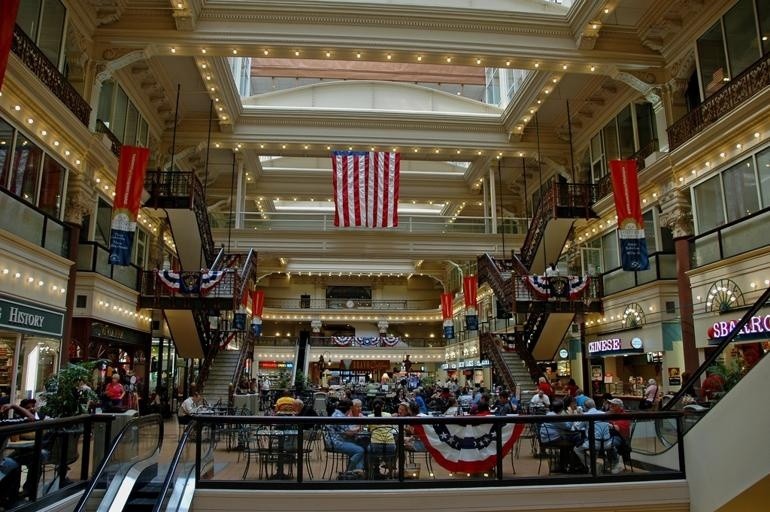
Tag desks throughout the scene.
[6,441,42,505]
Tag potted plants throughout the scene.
[39,354,111,461]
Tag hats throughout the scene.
[607,397,624,407]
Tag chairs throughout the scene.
[175,373,678,481]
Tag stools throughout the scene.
[19,460,56,499]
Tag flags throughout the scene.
[332,150,401,228]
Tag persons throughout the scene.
[643,378,658,403]
[105,373,126,406]
[292,398,322,440]
[681,372,697,397]
[542,261,560,278]
[228,374,271,410]
[177,391,202,424]
[274,388,297,414]
[9,398,47,502]
[0,397,36,509]
[698,367,727,403]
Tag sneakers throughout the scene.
[612,464,624,474]
